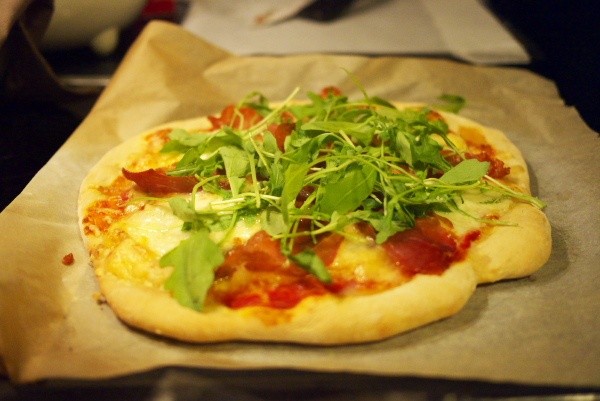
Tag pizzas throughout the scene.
[77,99,552,346]
